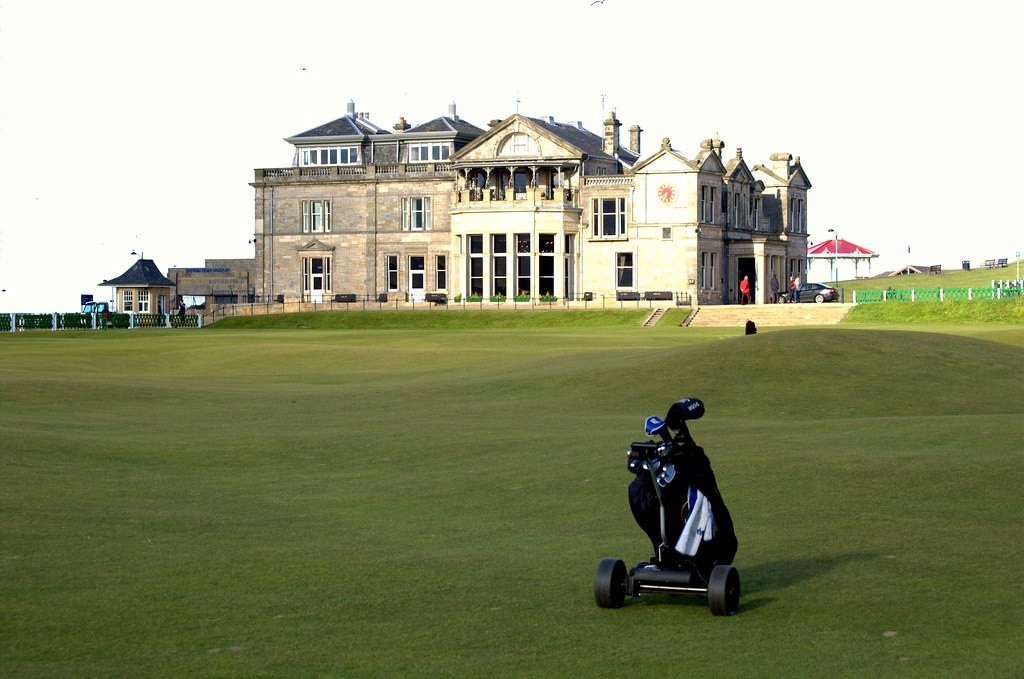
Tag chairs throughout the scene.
[273,295,284,303]
[375,294,387,302]
[581,292,592,301]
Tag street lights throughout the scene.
[828,229,839,302]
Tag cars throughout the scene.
[770,282,840,303]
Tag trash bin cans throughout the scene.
[962,261,970,271]
[277,295,284,304]
[249,293,255,303]
[379,293,387,302]
[584,292,592,301]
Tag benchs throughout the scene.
[616,291,640,300]
[332,294,356,302]
[424,293,447,304]
[645,292,672,300]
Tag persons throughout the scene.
[158,301,162,325]
[770,274,780,304]
[740,276,751,304]
[177,299,187,329]
[102,305,109,330]
[789,275,803,303]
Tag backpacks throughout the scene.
[745,321,757,335]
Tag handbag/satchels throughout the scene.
[629,443,738,589]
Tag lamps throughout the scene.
[249,239,256,243]
